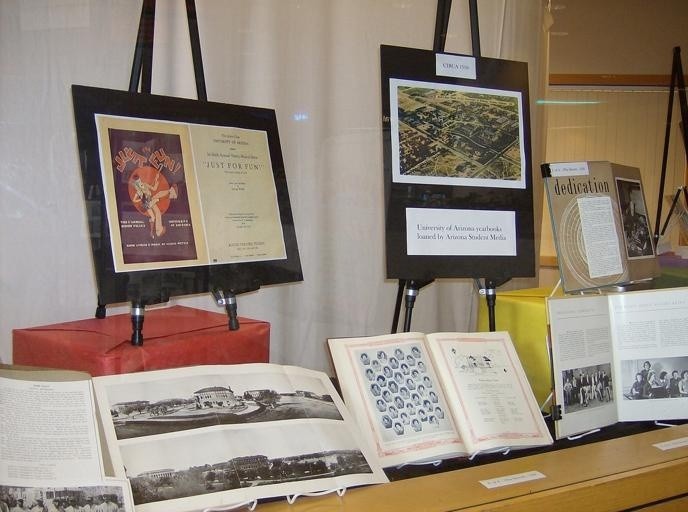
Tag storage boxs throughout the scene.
[12,305,271,378]
[476,285,615,415]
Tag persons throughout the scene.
[130,173,179,238]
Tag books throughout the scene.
[540,160,663,295]
[545,287,688,442]
[326,329,554,470]
[0,361,389,511]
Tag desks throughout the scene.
[229,424,688,512]
